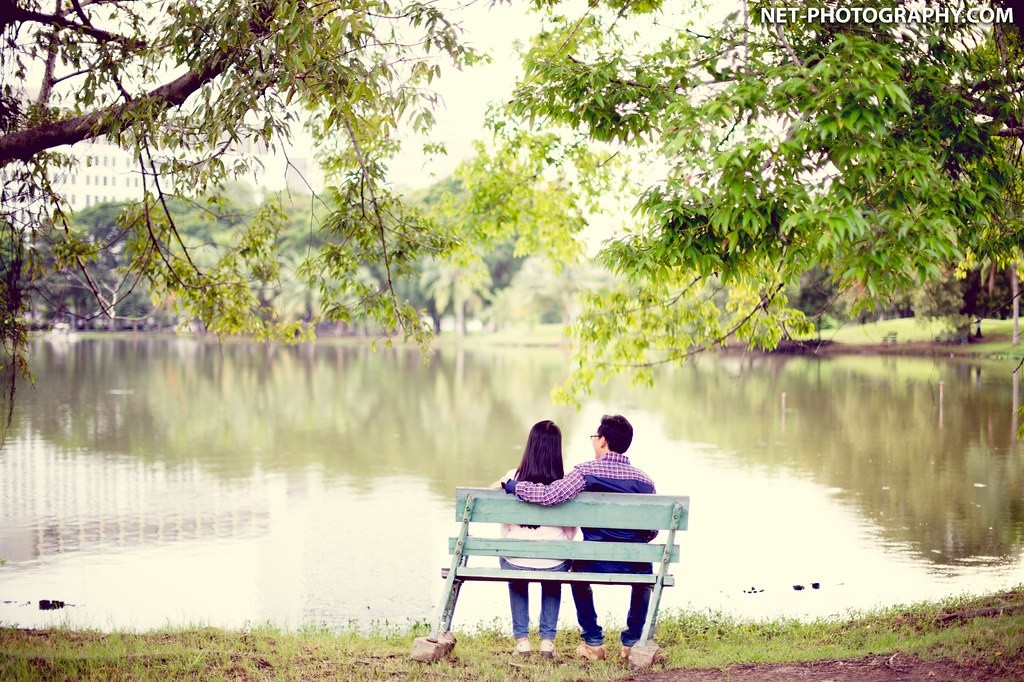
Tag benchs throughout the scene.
[424,487,689,645]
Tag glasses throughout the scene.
[590,435,601,441]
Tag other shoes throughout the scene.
[621,646,631,659]
[577,642,606,662]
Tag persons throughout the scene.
[489,420,584,658]
[493,414,660,660]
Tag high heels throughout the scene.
[540,640,557,660]
[511,641,530,662]
